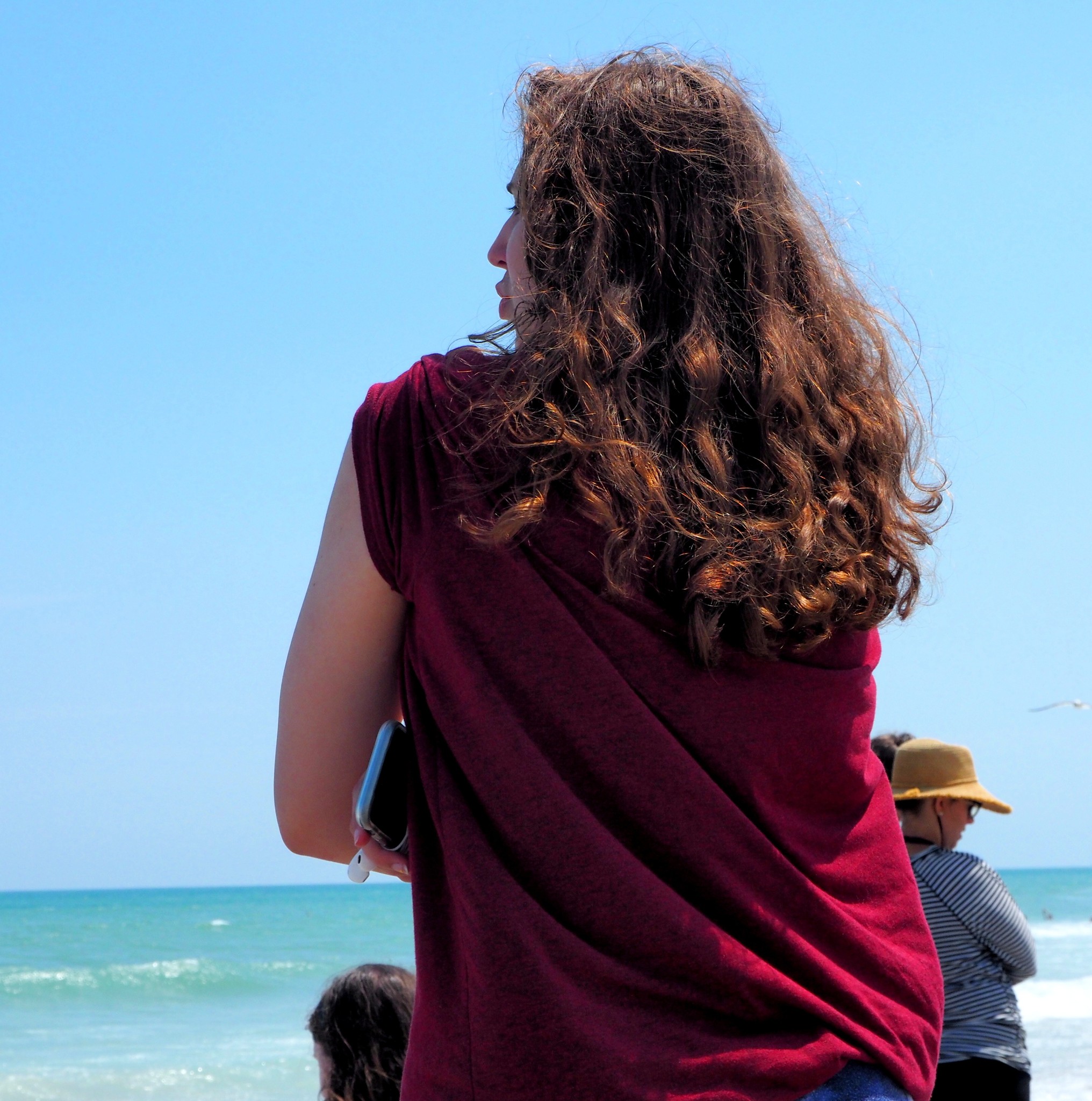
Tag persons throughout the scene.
[870,733,915,784]
[886,737,1036,1101]
[272,51,943,1100]
[305,964,418,1101]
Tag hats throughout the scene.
[889,738,1013,815]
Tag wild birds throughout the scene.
[1024,698,1092,714]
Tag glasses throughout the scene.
[966,800,983,817]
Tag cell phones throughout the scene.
[356,720,411,856]
